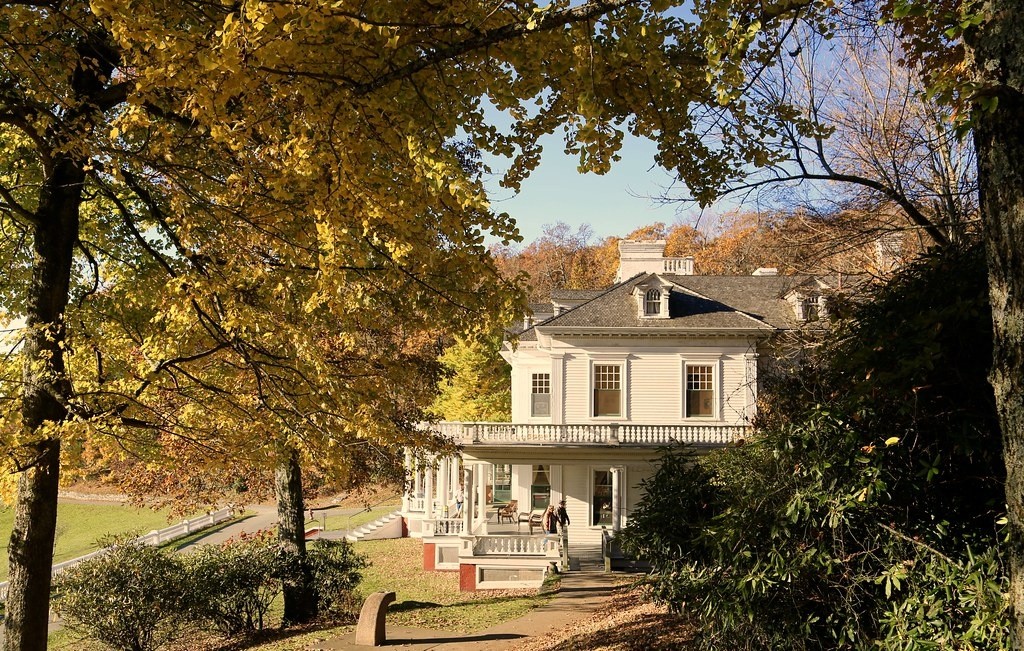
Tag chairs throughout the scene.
[498,499,518,524]
[517,508,547,534]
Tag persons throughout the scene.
[455,484,463,512]
[555,500,570,527]
[542,505,561,548]
